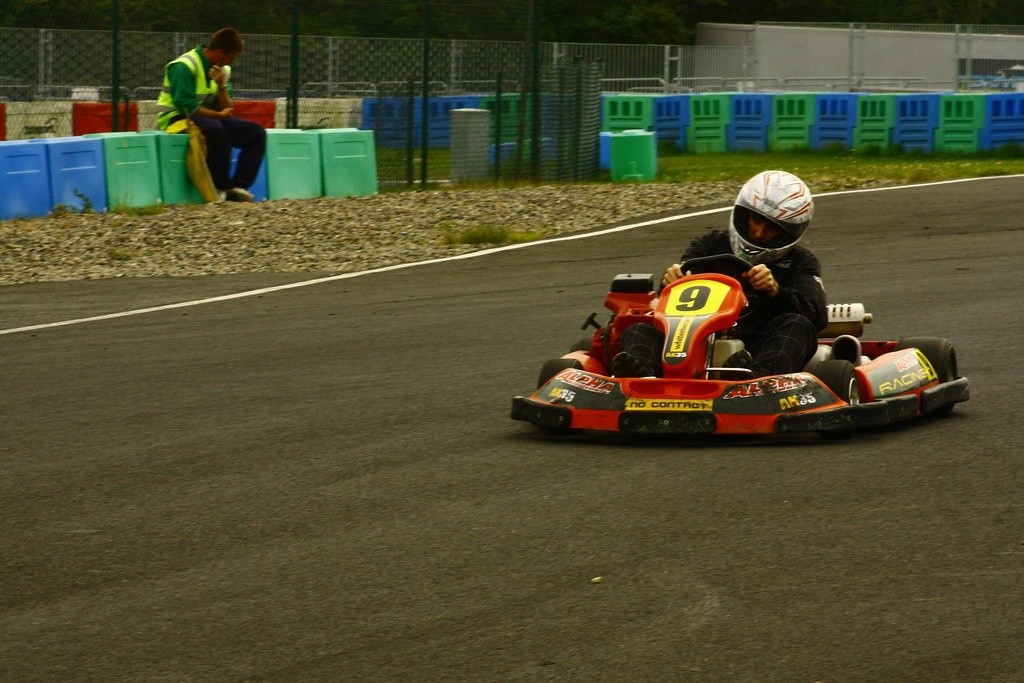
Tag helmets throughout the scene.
[729,170,814,265]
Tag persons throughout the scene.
[610,170,828,379]
[156,27,267,204]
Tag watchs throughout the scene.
[219,84,228,92]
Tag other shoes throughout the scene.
[611,352,644,378]
[720,349,752,380]
[214,192,226,203]
[228,187,253,202]
